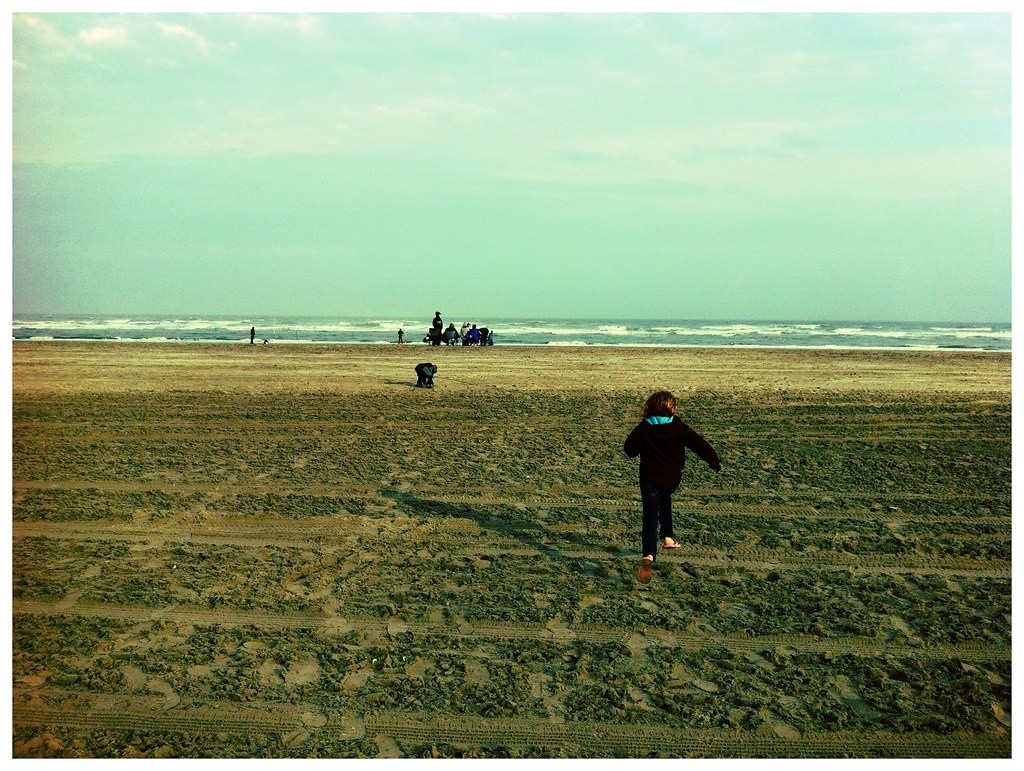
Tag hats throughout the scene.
[467,323,470,325]
[436,312,441,314]
[463,322,467,327]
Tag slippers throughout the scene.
[661,540,681,549]
[636,557,653,584]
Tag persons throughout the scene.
[264,340,268,344]
[623,391,721,584]
[424,312,493,346]
[398,329,404,344]
[415,363,437,388]
[250,327,255,344]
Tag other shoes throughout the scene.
[426,383,433,387]
[416,381,423,386]
